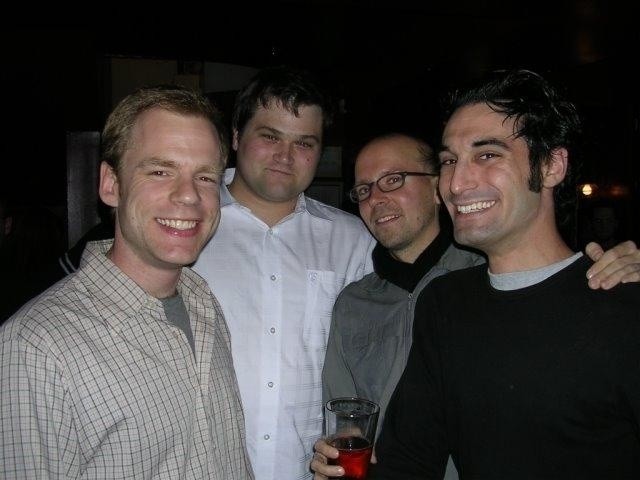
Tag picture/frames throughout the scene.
[304,180,348,213]
[315,141,344,179]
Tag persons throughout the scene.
[320,125,639,480]
[0,86,254,480]
[191,71,377,480]
[310,67,639,480]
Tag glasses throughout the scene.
[347,171,432,203]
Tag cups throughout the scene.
[323,396,380,480]
